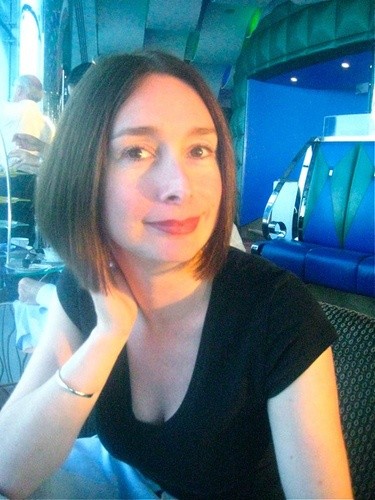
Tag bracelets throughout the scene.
[38,157,44,167]
[54,369,95,398]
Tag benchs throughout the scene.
[250,134,375,300]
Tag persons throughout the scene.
[0,50,353,500]
[3,74,56,223]
[7,62,95,169]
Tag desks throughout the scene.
[4,253,71,305]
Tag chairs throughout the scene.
[295,298,375,499]
